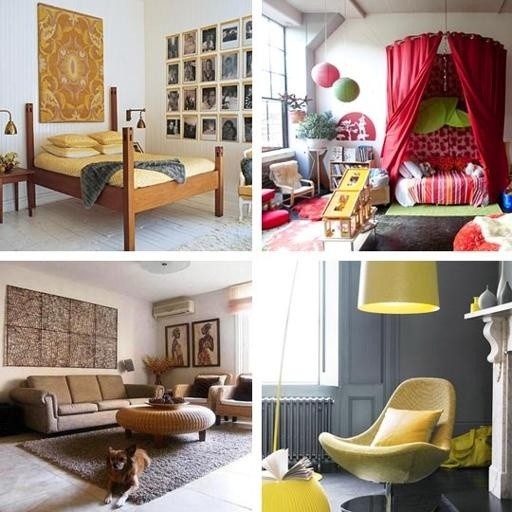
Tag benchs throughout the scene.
[267,159,312,210]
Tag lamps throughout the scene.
[0,109,17,136]
[310,0,340,89]
[356,261,440,316]
[332,0,360,103]
[126,107,146,128]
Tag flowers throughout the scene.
[141,353,173,374]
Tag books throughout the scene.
[262,446,313,483]
[328,144,370,189]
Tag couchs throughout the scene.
[215,373,252,424]
[174,372,232,421]
[11,374,165,435]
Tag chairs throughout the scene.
[239,148,252,222]
[318,377,456,512]
[366,170,391,207]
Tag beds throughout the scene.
[24,88,224,251]
[380,32,508,206]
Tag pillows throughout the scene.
[90,131,122,143]
[41,144,101,159]
[193,377,220,398]
[94,145,127,154]
[234,377,252,401]
[397,165,411,179]
[47,134,99,148]
[371,408,442,449]
[404,160,422,178]
[240,157,251,186]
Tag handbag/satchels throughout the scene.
[440,426,492,468]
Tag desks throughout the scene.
[262,466,330,512]
[0,167,36,224]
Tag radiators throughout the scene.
[263,396,335,471]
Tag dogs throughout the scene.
[103,443,151,507]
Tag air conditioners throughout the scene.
[153,300,195,318]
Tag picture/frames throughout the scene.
[133,142,142,153]
[192,318,221,367]
[165,323,190,369]
[165,15,253,145]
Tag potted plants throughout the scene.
[279,93,312,122]
[294,111,338,149]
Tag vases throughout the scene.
[155,375,162,385]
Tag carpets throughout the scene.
[290,196,329,222]
[358,213,485,251]
[20,422,253,505]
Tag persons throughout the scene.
[197,323,215,364]
[169,326,185,365]
[167,19,251,143]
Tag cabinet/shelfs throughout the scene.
[330,159,374,192]
[318,168,379,250]
